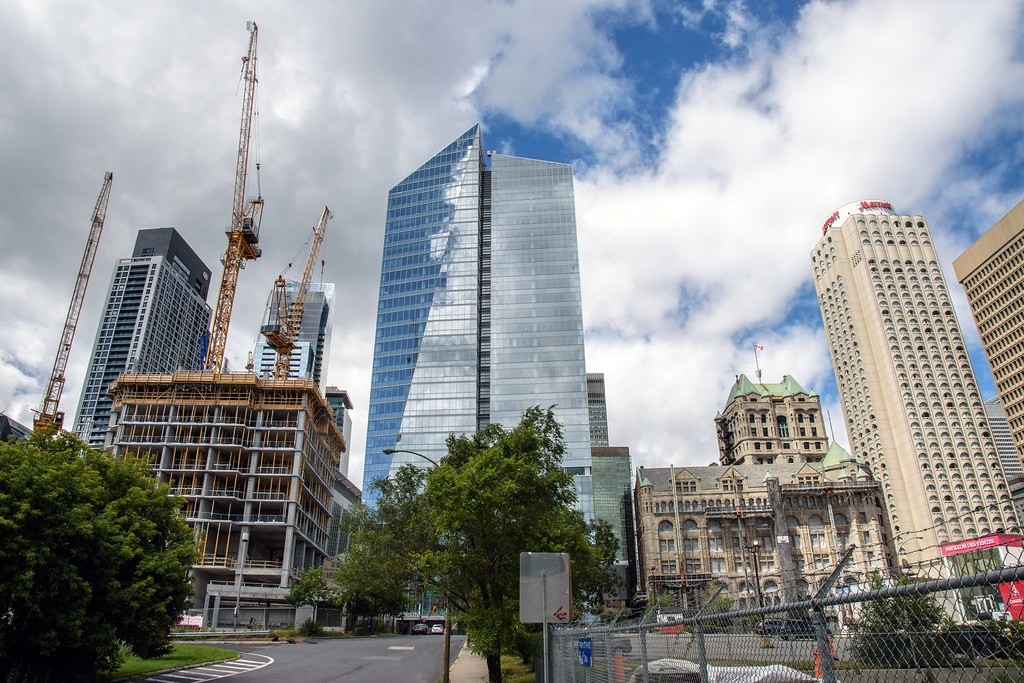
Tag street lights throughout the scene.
[741,531,775,648]
[382,447,452,683]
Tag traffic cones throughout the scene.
[828,636,839,660]
[815,652,825,683]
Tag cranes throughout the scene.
[260,205,334,380]
[198,18,266,374]
[27,171,117,436]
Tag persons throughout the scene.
[810,629,836,683]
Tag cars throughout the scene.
[412,623,429,634]
[431,624,445,635]
[566,636,632,657]
[783,619,834,640]
[755,619,785,635]
